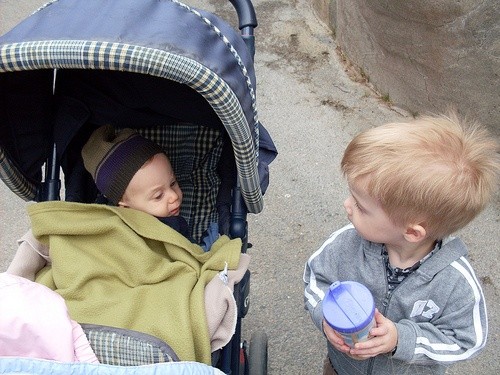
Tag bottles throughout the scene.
[323,281,377,360]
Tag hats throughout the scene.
[81,123,164,205]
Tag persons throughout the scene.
[300,103,500,375]
[53,121,199,366]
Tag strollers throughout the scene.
[0,0,279,375]
